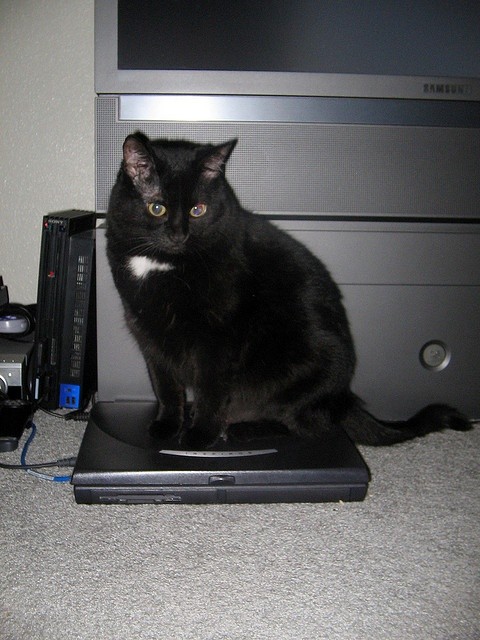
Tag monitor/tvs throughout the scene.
[93,1,480,407]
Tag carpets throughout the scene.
[0,400,480,640]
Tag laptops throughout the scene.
[65,403,373,506]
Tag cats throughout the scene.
[103,128,475,453]
